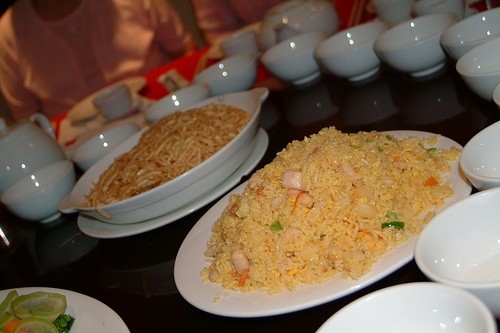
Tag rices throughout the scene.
[199,126,461,302]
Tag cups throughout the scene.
[221,31,260,60]
[373,0,465,23]
[92,84,132,118]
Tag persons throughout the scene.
[0,0,194,129]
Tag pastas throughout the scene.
[83,102,250,219]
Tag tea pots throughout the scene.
[1,113,63,191]
[257,0,339,51]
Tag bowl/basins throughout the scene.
[440,7,499,60]
[260,32,325,85]
[374,14,456,78]
[58,88,270,223]
[193,54,258,92]
[1,161,74,224]
[72,122,138,172]
[144,82,211,121]
[459,120,500,192]
[315,282,498,333]
[312,18,387,81]
[456,35,499,106]
[415,186,500,316]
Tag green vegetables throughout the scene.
[53,314,75,333]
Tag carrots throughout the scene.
[5,320,28,333]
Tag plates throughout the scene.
[77,127,269,239]
[0,288,129,333]
[67,76,147,117]
[173,130,472,317]
